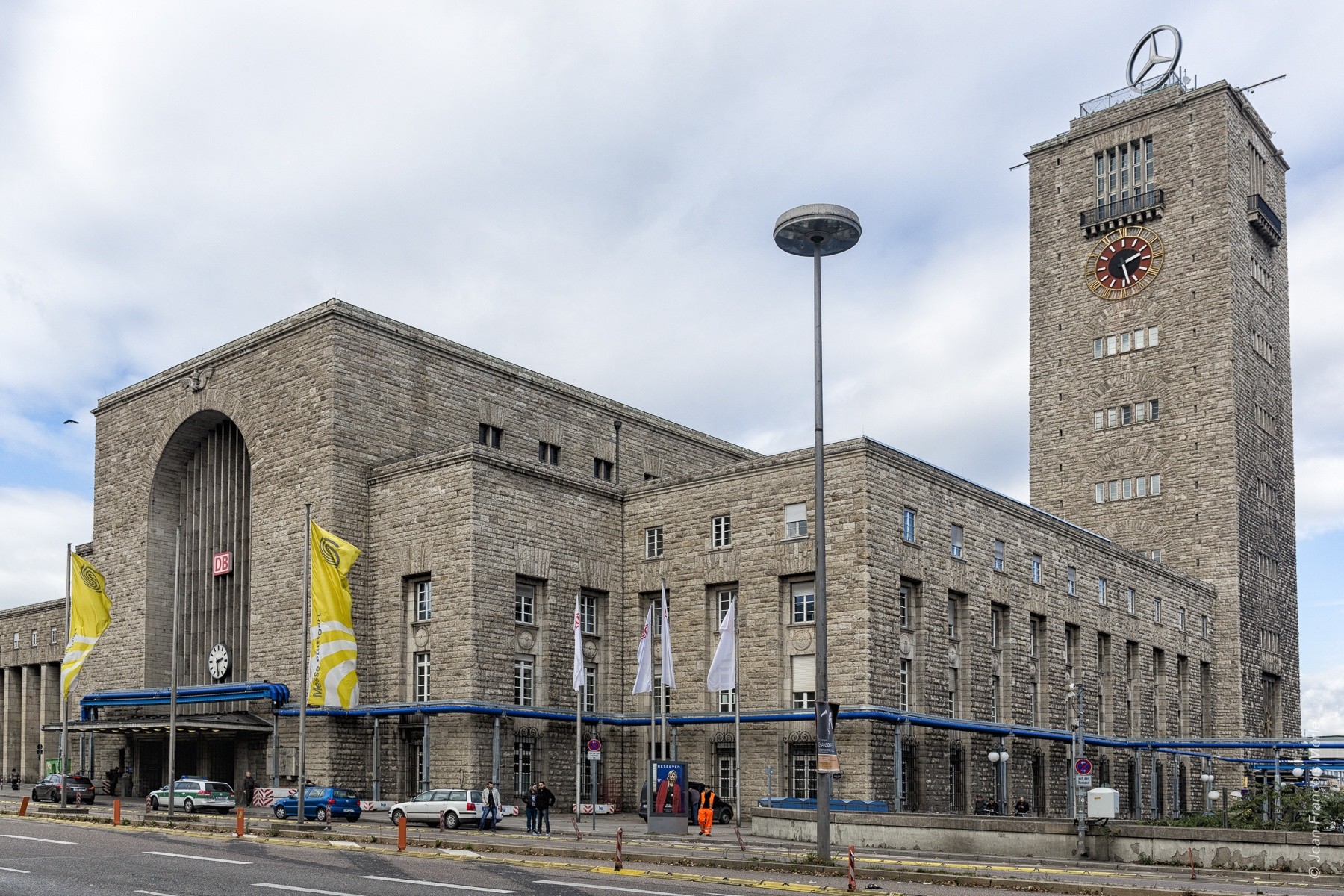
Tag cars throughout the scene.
[273,786,361,822]
[148,774,235,813]
[387,789,504,830]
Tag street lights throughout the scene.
[1199,760,1220,816]
[774,203,864,861]
[1293,767,1324,791]
[1207,787,1242,828]
[985,741,1010,815]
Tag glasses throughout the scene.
[533,786,537,789]
[539,784,544,786]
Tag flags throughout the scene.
[305,518,361,710]
[572,593,585,693]
[60,551,113,702]
[706,596,736,691]
[631,604,653,695]
[661,582,677,689]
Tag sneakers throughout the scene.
[532,830,537,836]
[491,829,496,833]
[536,831,542,835]
[707,834,712,837]
[478,830,484,834]
[699,831,704,835]
[546,832,550,836]
[525,829,531,834]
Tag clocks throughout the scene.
[1082,225,1164,300]
[207,644,232,680]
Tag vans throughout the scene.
[638,780,733,825]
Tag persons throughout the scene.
[108,767,121,797]
[477,781,503,834]
[973,793,1029,817]
[533,781,555,836]
[305,779,315,787]
[689,788,701,825]
[697,785,717,837]
[242,771,260,809]
[522,783,538,834]
[656,770,682,813]
[10,769,21,790]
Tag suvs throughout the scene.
[31,772,95,804]
